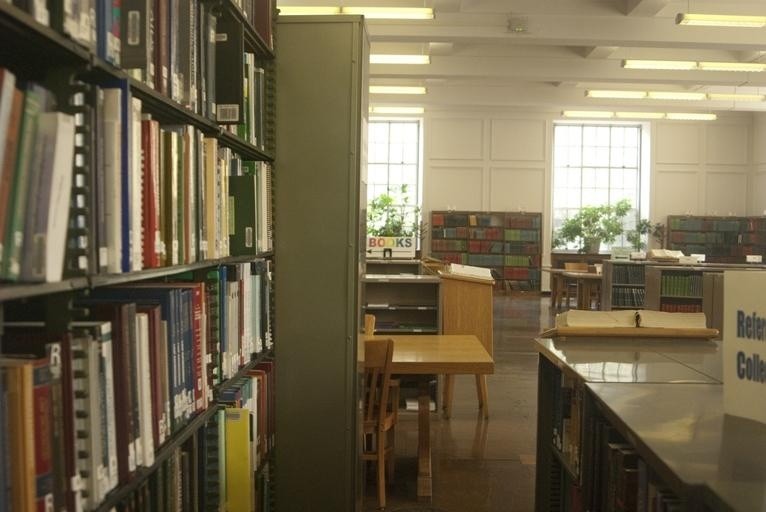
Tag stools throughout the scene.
[443,374,488,419]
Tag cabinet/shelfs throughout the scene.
[601,261,766,311]
[432,211,542,290]
[667,215,766,264]
[534,339,766,512]
[362,274,442,416]
[646,266,766,313]
[0,0,275,512]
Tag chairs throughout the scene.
[365,314,399,425]
[565,262,587,307]
[364,340,395,509]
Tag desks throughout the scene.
[562,272,602,310]
[542,268,588,308]
[422,260,496,362]
[359,334,493,505]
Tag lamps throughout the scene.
[561,0,766,120]
[276,0,435,115]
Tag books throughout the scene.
[432,214,540,291]
[367,300,436,411]
[612,216,766,312]
[1,0,275,150]
[1,71,274,283]
[547,366,688,512]
[0,257,275,511]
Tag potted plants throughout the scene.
[552,199,632,254]
